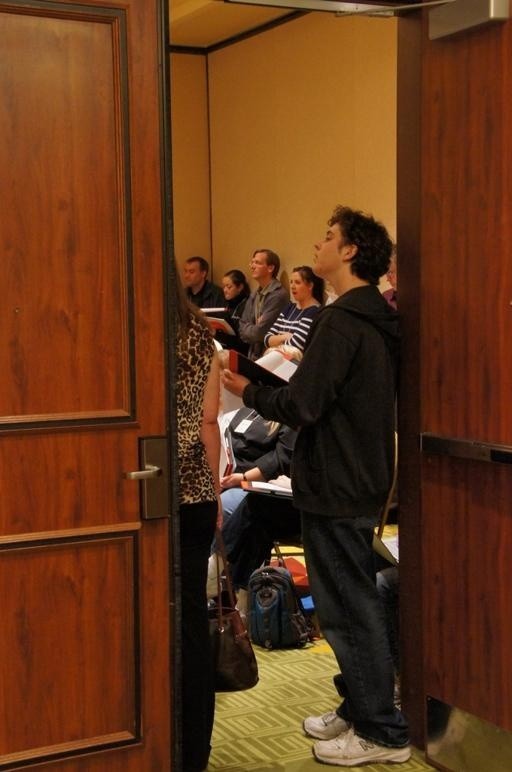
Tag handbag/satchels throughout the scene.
[207,608,259,693]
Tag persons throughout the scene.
[211,203,417,768]
[169,258,224,770]
[384,250,398,308]
[185,250,325,596]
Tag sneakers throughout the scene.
[206,571,224,599]
[311,728,412,767]
[302,710,354,740]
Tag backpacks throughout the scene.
[247,565,311,650]
